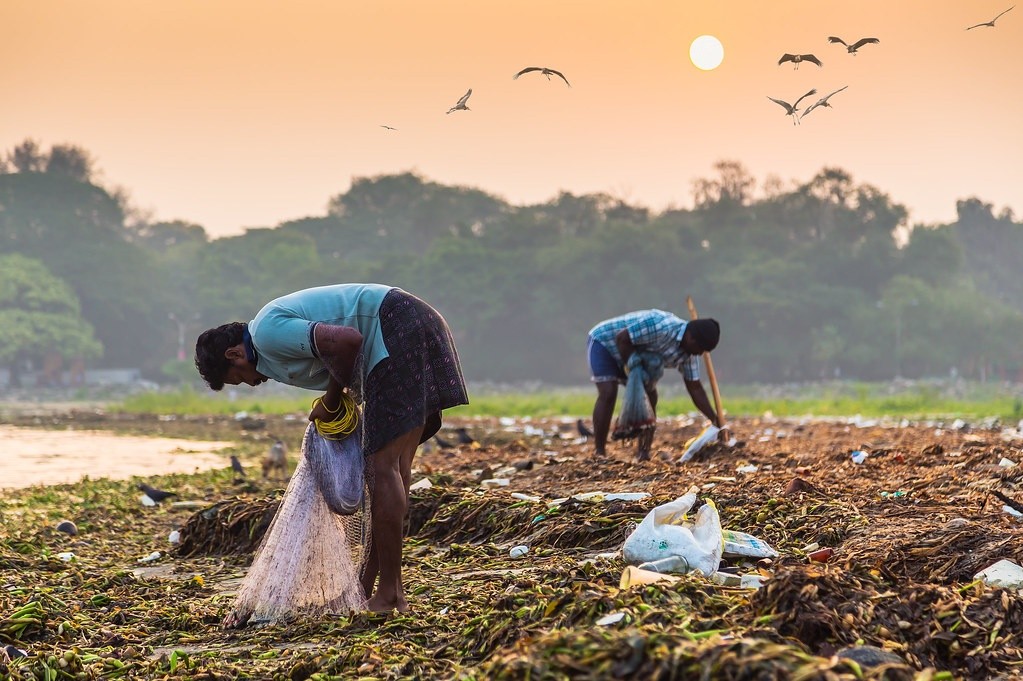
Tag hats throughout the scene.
[688,318,721,352]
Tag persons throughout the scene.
[194,282,470,619]
[586,307,730,462]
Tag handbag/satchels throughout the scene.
[623,489,725,579]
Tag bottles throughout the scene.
[639,555,690,576]
[800,548,834,565]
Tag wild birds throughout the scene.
[767,86,849,125]
[230,455,247,481]
[828,35,880,56]
[139,482,178,506]
[381,124,397,132]
[446,89,473,114]
[578,419,594,443]
[434,427,473,447]
[514,67,572,88]
[963,5,1015,30]
[778,53,824,71]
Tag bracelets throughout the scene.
[319,393,342,414]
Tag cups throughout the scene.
[618,565,684,593]
